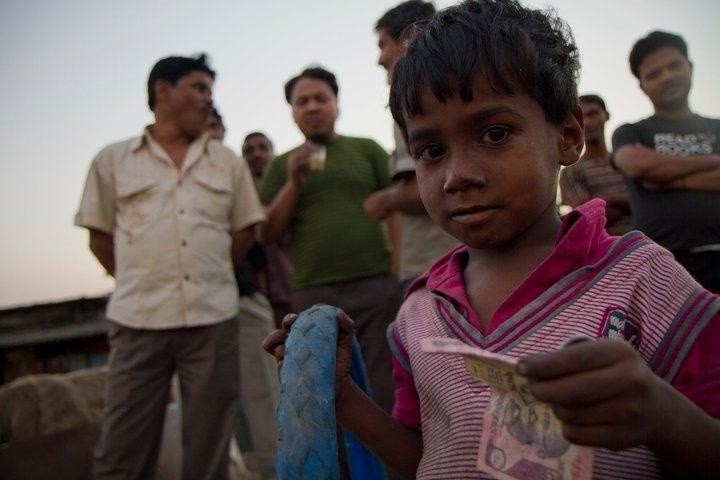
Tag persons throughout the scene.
[262,0,720,480]
[259,64,403,418]
[363,1,462,302]
[170,109,256,480]
[242,132,275,185]
[611,30,720,293]
[560,94,632,229]
[74,55,268,480]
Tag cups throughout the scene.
[306,146,325,171]
